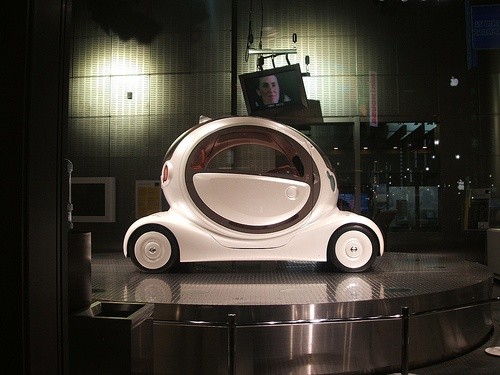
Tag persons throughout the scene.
[254,74,291,107]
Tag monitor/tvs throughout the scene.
[238,63,308,118]
[71,176,116,222]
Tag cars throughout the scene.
[124,115,385,275]
